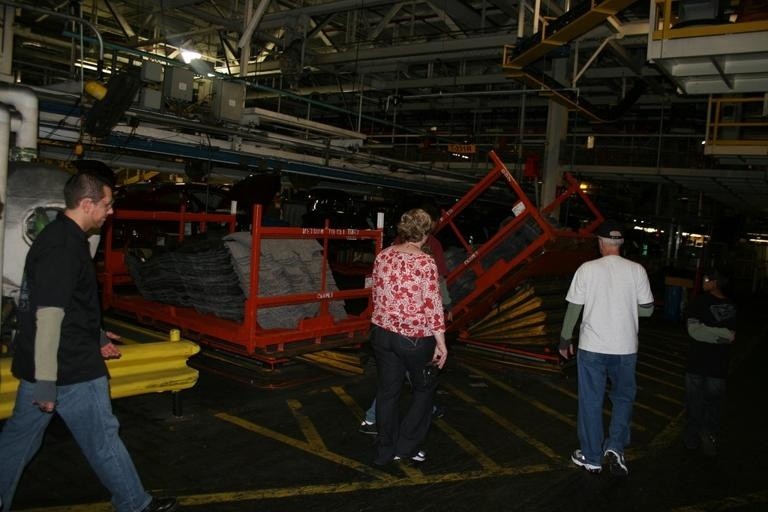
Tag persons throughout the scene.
[1,166,180,512]
[682,264,740,459]
[370,207,449,465]
[557,218,657,476]
[360,206,448,436]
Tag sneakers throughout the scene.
[358,420,378,435]
[686,431,722,451]
[412,450,427,465]
[142,496,177,512]
[571,449,603,473]
[604,449,628,478]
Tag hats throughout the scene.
[590,221,625,240]
